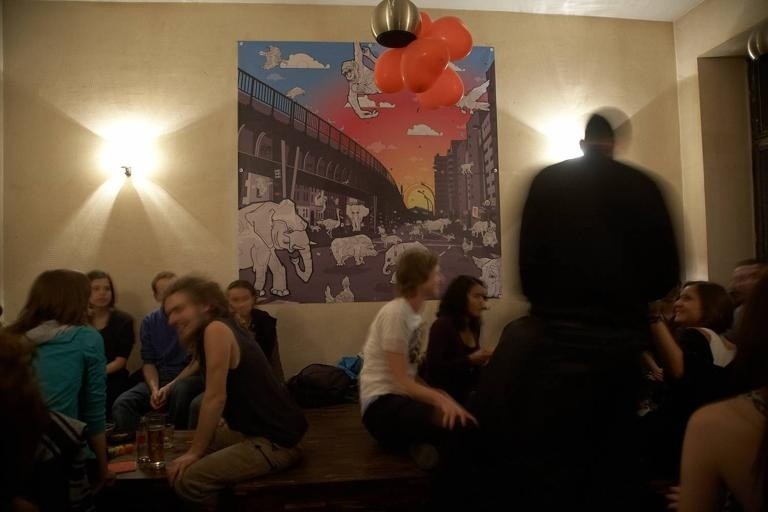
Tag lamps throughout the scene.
[371,0,423,50]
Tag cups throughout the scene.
[135,413,175,471]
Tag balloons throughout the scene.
[374,10,473,110]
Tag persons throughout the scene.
[634,256,768,512]
[362,251,530,512]
[0,268,308,512]
[517,109,682,512]
